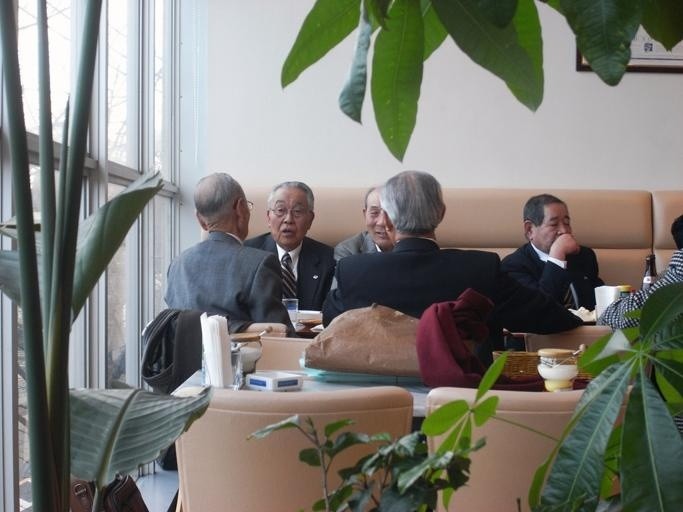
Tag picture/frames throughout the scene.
[573,22,682,71]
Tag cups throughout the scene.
[199,352,241,390]
[227,332,263,377]
[594,304,608,320]
[536,348,579,392]
[280,299,298,320]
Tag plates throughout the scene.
[297,319,321,327]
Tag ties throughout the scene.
[281,254,296,298]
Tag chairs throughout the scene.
[159,382,415,512]
[423,384,626,512]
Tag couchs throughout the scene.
[648,190,682,275]
[196,181,653,295]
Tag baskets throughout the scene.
[491,351,593,382]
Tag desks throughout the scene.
[164,320,629,418]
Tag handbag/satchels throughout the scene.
[305,305,419,375]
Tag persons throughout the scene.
[595,210,682,439]
[163,173,305,338]
[331,185,396,290]
[241,179,335,312]
[498,193,605,324]
[319,169,585,371]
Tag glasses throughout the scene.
[272,206,305,216]
[243,200,252,210]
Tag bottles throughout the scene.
[640,254,658,291]
[501,331,522,352]
[617,285,632,299]
